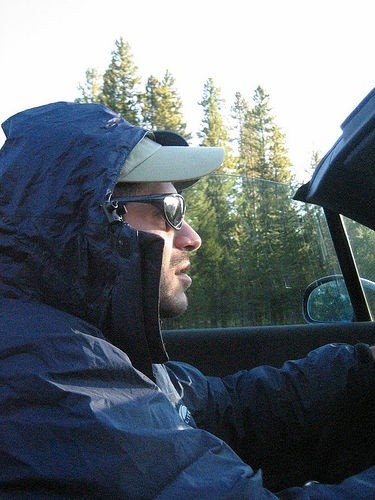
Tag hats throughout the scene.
[118,138,225,190]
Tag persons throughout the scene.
[0,100,375,500]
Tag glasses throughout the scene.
[119,192,185,230]
